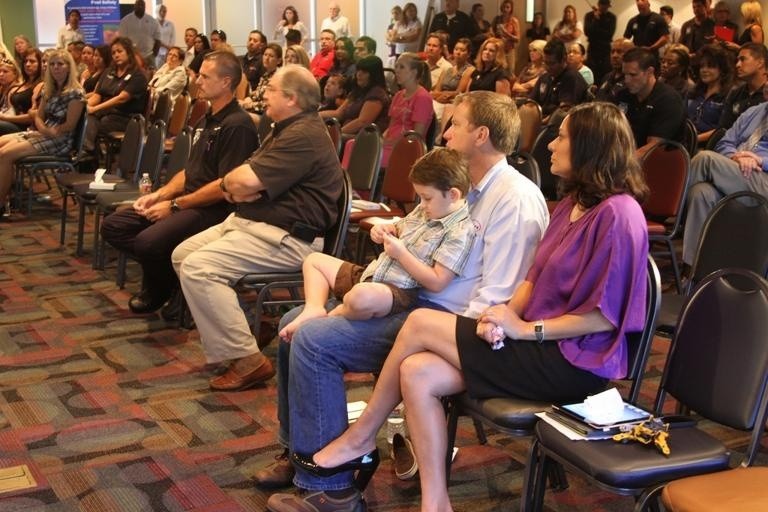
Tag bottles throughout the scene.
[386,402,405,445]
[138,173,152,199]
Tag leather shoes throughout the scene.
[161,288,182,318]
[208,356,276,392]
[250,321,276,351]
[128,288,171,313]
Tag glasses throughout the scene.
[262,85,285,93]
[543,60,558,66]
[217,28,221,35]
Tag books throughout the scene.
[546,409,621,437]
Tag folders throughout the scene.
[542,405,667,437]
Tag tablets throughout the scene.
[552,397,655,429]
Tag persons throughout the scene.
[705,2,739,46]
[291,101,647,512]
[527,38,595,102]
[680,0,714,49]
[120,1,159,56]
[254,91,550,511]
[619,46,688,139]
[330,35,355,71]
[286,45,310,66]
[251,43,283,115]
[555,4,584,49]
[375,51,433,167]
[172,63,345,391]
[209,29,232,51]
[317,71,351,111]
[725,1,763,52]
[385,6,403,60]
[309,29,337,79]
[619,42,619,45]
[427,0,472,48]
[685,46,732,150]
[345,33,377,75]
[284,27,303,45]
[241,29,268,93]
[720,42,768,139]
[589,38,637,104]
[466,3,493,37]
[466,37,511,93]
[320,56,390,134]
[67,39,85,67]
[273,5,308,46]
[512,42,549,101]
[416,32,452,87]
[0,50,86,210]
[82,45,113,99]
[623,0,666,53]
[187,33,207,68]
[660,44,695,102]
[78,36,149,168]
[213,43,235,55]
[277,146,476,344]
[39,48,55,74]
[11,33,34,55]
[153,4,176,50]
[320,1,352,37]
[390,3,422,62]
[79,44,96,87]
[101,50,256,313]
[182,29,200,48]
[492,0,520,80]
[57,9,86,57]
[523,12,550,40]
[430,38,474,99]
[662,72,767,296]
[146,46,188,113]
[567,42,594,88]
[659,5,680,54]
[583,0,618,69]
[0,53,23,112]
[0,47,43,133]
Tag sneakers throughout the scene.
[393,433,418,480]
[266,488,368,512]
[254,453,293,486]
[72,148,96,161]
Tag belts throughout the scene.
[316,230,326,237]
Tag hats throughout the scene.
[598,0,611,7]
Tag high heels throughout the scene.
[292,447,381,492]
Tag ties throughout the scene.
[736,115,768,152]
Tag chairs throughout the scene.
[633,141,690,296]
[344,131,428,265]
[325,117,342,158]
[532,267,768,512]
[11,98,87,216]
[662,384,768,512]
[56,114,132,255]
[78,113,145,266]
[120,125,194,289]
[446,255,660,512]
[384,67,399,96]
[508,152,540,193]
[347,124,382,261]
[237,170,352,347]
[516,97,542,156]
[653,191,768,337]
[99,119,166,269]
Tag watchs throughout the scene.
[533,318,545,345]
[169,198,178,214]
